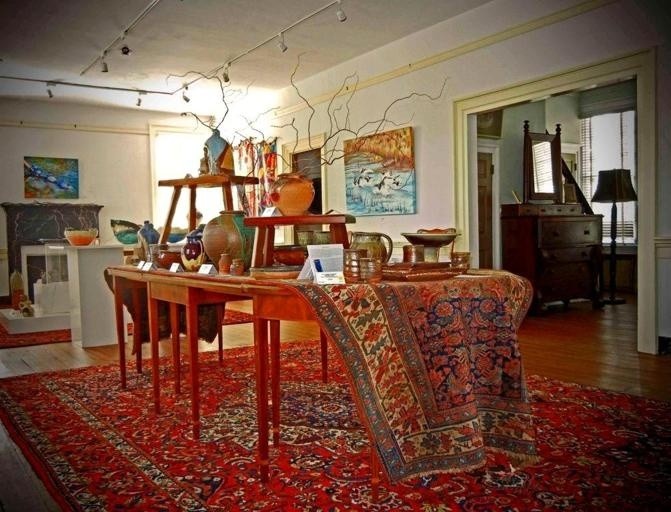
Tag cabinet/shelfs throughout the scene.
[502,203,602,317]
[67,246,128,349]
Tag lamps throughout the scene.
[46,83,145,106]
[591,169,637,304]
[100,33,130,72]
[183,3,347,102]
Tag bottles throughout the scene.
[229,258,244,274]
[217,253,231,275]
[124,251,134,265]
[9,268,23,310]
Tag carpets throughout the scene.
[0,308,254,348]
[0,339,671,512]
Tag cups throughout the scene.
[453,252,470,273]
[360,258,381,283]
[342,247,367,276]
[401,245,425,263]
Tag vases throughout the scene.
[138,130,315,272]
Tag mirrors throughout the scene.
[523,120,562,205]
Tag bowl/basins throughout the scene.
[273,244,305,264]
[160,250,180,269]
[65,230,96,246]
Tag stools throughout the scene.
[245,215,356,267]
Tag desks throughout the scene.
[103,266,540,502]
